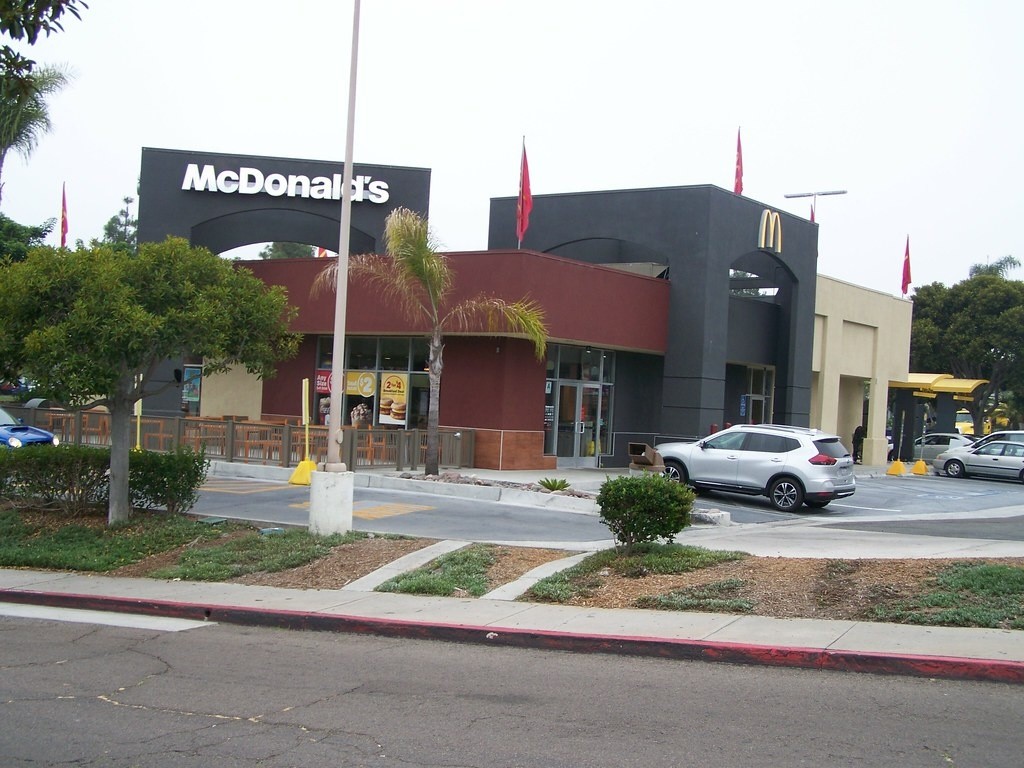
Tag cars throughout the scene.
[0,407,61,453]
[933,440,1024,484]
[885,403,1024,463]
[0,374,42,396]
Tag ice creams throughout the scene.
[351,404,372,429]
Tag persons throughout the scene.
[852,424,867,465]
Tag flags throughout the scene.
[60,181,68,248]
[811,207,814,222]
[901,233,912,294]
[515,145,533,241]
[735,124,743,195]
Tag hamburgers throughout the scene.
[379,397,393,415]
[390,402,406,419]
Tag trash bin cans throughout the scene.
[24,398,50,431]
[628,443,665,480]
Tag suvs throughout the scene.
[652,423,856,513]
